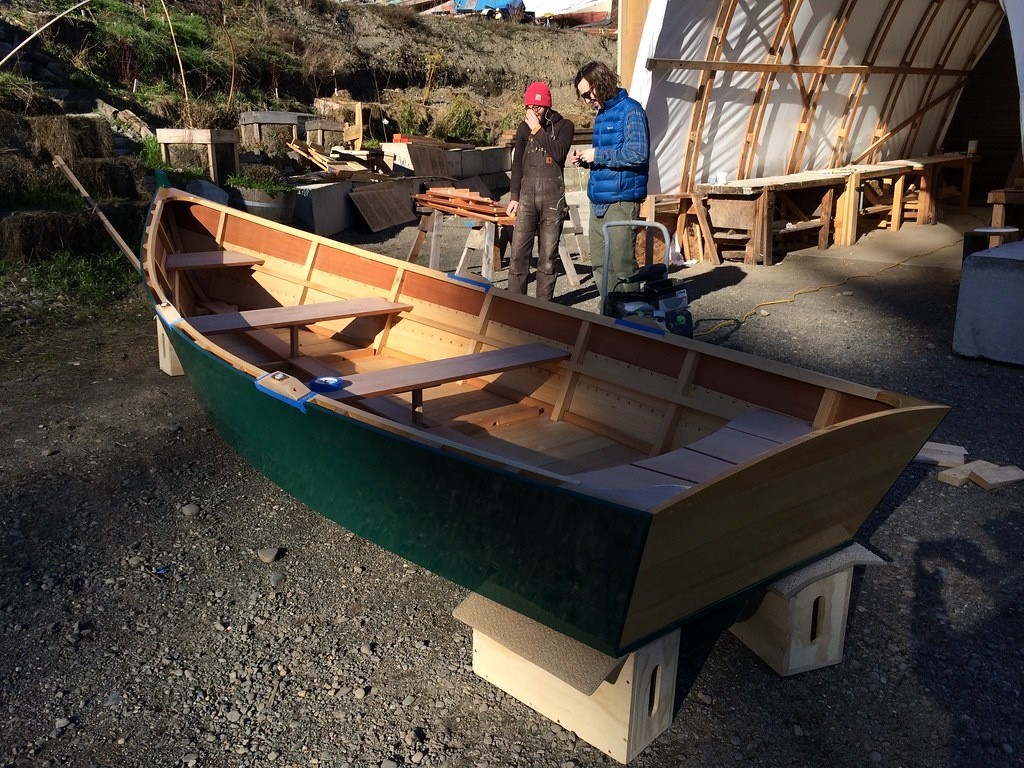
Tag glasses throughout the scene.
[581,85,596,99]
[525,106,540,112]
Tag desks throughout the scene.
[406,193,588,286]
[986,187,1024,248]
[696,151,978,266]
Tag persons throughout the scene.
[570,62,651,298]
[505,82,575,302]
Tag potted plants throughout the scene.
[361,139,384,173]
[223,164,298,224]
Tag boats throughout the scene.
[137,166,953,659]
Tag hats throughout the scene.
[524,82,552,107]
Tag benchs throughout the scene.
[183,296,413,358]
[302,343,572,425]
[165,249,265,270]
[567,410,820,509]
